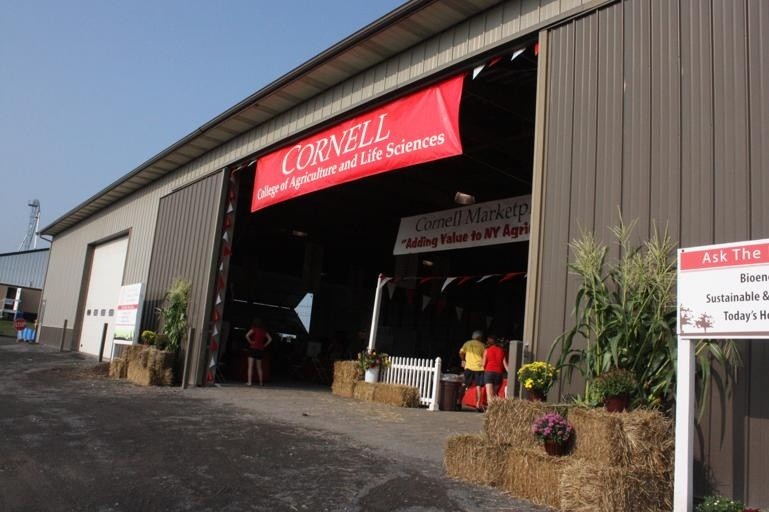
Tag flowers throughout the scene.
[532,412,571,443]
[359,349,388,369]
[517,362,559,392]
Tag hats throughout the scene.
[471,330,483,339]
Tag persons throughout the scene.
[455,329,486,412]
[244,317,273,387]
[481,337,508,408]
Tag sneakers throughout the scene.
[476,404,484,412]
[456,403,461,410]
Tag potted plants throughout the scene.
[592,369,640,412]
[140,330,155,346]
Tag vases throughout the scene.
[545,439,570,454]
[527,391,547,401]
[364,366,380,383]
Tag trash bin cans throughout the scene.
[440,373,465,411]
